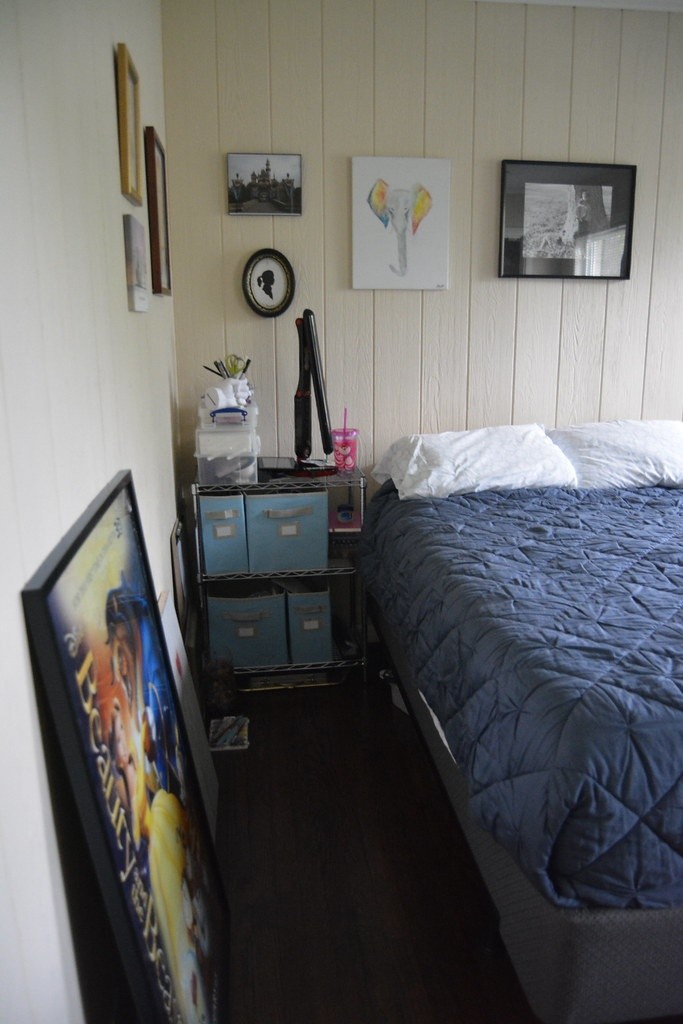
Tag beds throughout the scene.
[359,482,683,1024]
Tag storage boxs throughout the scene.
[206,579,289,667]
[194,427,257,456]
[194,435,261,486]
[286,578,333,664]
[243,483,329,574]
[197,395,259,429]
[198,488,250,576]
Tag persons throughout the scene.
[574,191,593,239]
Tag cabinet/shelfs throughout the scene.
[191,465,369,699]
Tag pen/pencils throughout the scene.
[201,359,251,380]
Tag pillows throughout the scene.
[370,423,578,499]
[546,420,683,490]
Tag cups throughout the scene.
[333,429,358,471]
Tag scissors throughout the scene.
[224,353,246,378]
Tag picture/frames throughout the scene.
[242,247,295,318]
[117,42,144,207]
[144,125,172,298]
[227,152,302,217]
[498,159,638,280]
[19,469,233,1024]
